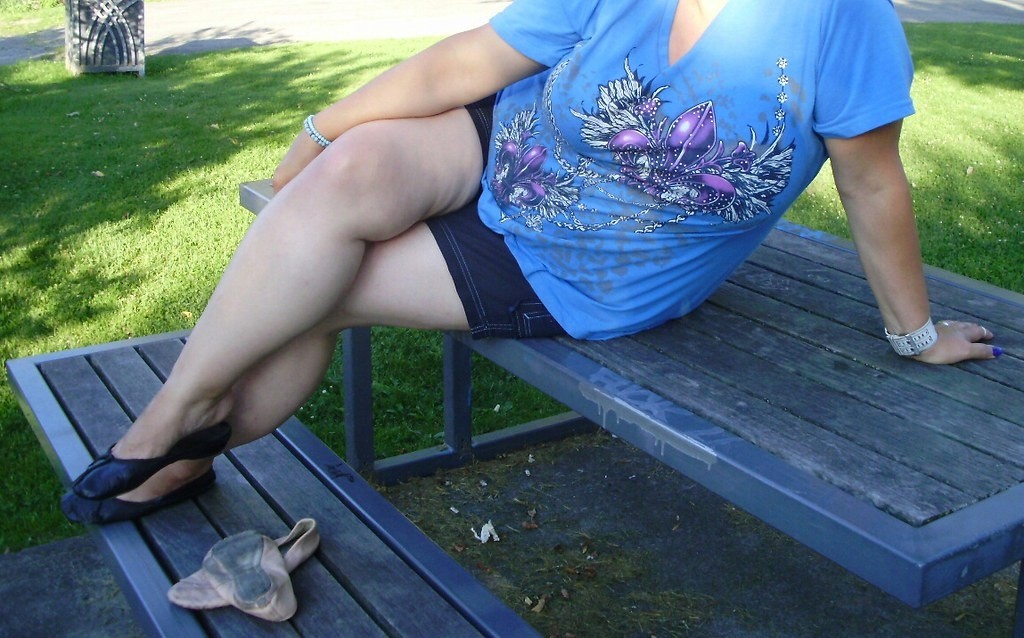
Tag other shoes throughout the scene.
[72,422,234,500]
[166,516,320,610]
[60,467,218,521]
[201,532,296,620]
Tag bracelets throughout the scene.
[304,118,327,149]
[308,115,332,146]
[885,318,939,356]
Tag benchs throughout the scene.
[239,171,1023,612]
[4,328,550,638]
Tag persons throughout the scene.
[51,0,1005,534]
